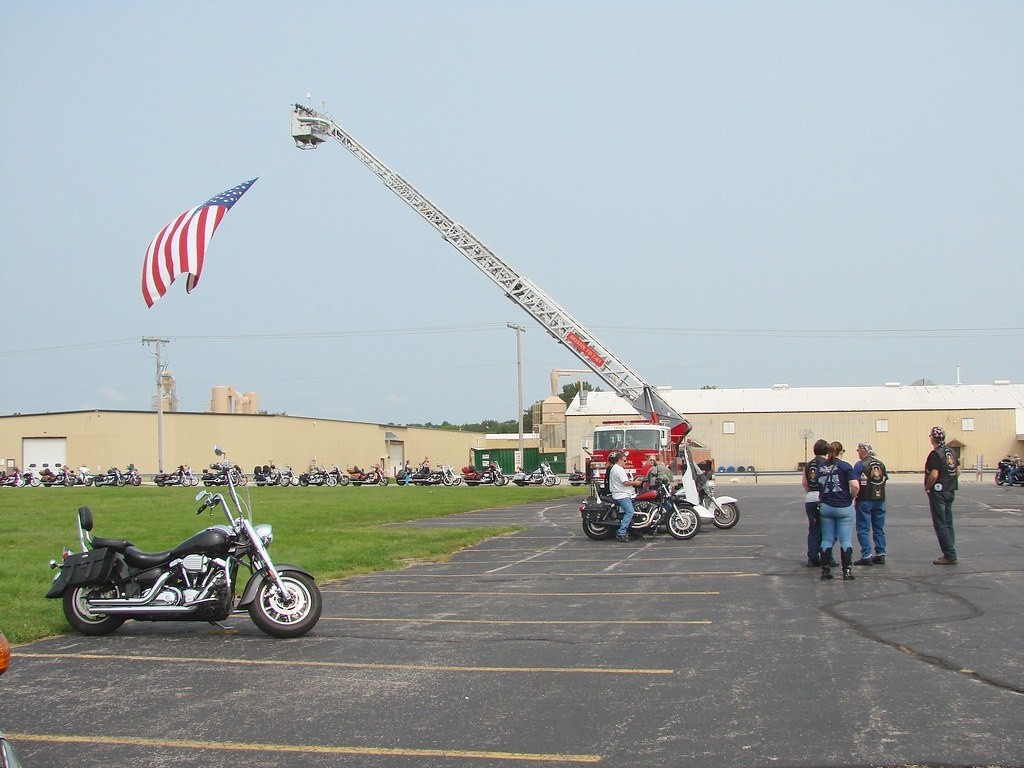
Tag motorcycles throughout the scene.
[43,442,323,638]
[251,461,350,487]
[346,464,390,487]
[153,463,199,488]
[578,453,740,541]
[512,457,562,487]
[200,461,248,487]
[0,462,143,489]
[568,468,588,487]
[393,460,510,486]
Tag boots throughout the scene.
[820,547,833,580]
[840,547,856,580]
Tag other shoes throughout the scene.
[819,562,839,569]
[808,558,820,567]
[615,535,629,542]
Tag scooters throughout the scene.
[995,455,1024,488]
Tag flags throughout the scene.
[142,177,259,309]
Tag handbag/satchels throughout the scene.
[812,504,820,525]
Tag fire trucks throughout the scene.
[291,93,718,490]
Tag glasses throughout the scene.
[928,435,932,439]
[841,449,845,453]
[621,458,627,462]
[856,449,859,452]
[649,459,655,461]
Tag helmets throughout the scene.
[609,452,618,458]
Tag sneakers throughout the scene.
[932,555,958,565]
[853,553,874,566]
[872,552,886,564]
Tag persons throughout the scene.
[605,452,616,496]
[640,456,656,476]
[924,427,960,565]
[1006,455,1023,486]
[609,453,643,542]
[852,442,889,565]
[802,439,838,567]
[816,442,859,580]
